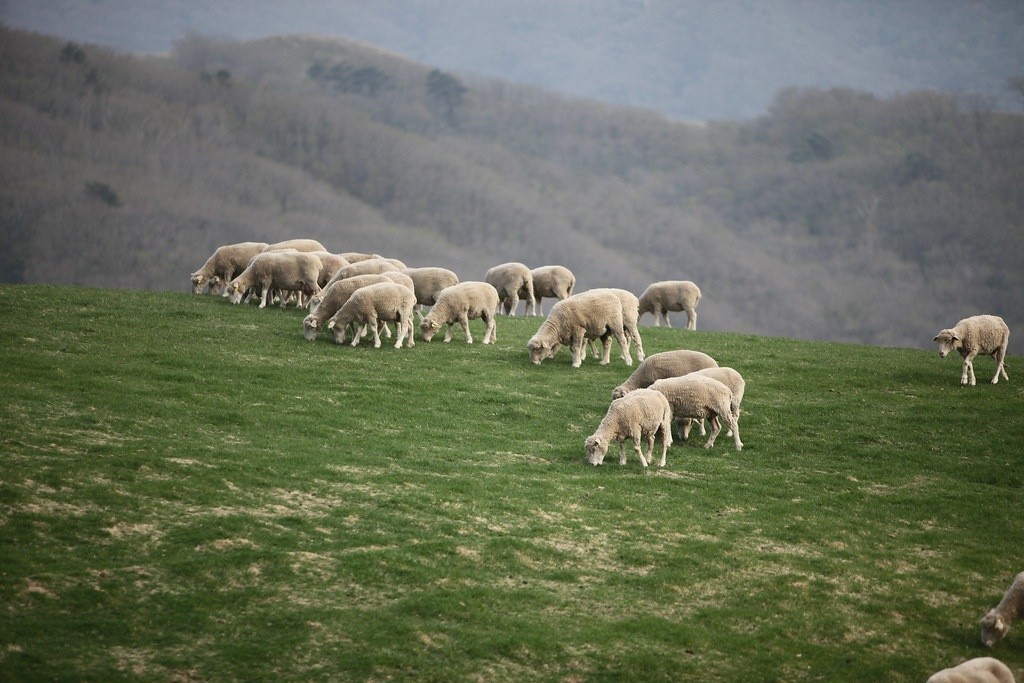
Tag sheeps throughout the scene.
[192,238,500,351]
[638,280,702,330]
[932,314,1009,386]
[926,571,1023,683]
[484,261,577,317]
[526,287,645,368]
[583,349,746,469]
